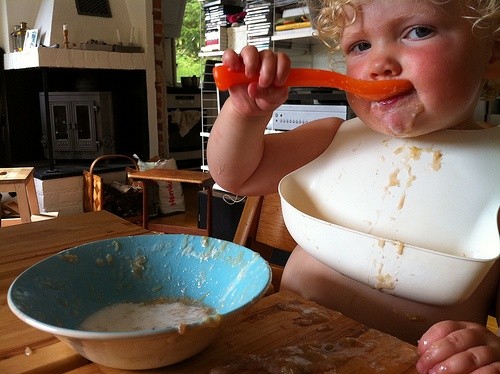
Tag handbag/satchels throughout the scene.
[131,153,188,215]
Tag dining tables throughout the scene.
[0,211,420,374]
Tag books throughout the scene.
[243,2,272,52]
[200,0,228,52]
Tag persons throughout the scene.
[206,0,500,374]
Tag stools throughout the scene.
[0,167,40,228]
[128,169,216,237]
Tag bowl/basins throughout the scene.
[6,233,273,371]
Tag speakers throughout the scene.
[198,190,246,243]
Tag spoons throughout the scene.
[213,63,415,102]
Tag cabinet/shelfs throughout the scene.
[198,0,317,170]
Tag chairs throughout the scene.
[232,192,297,293]
